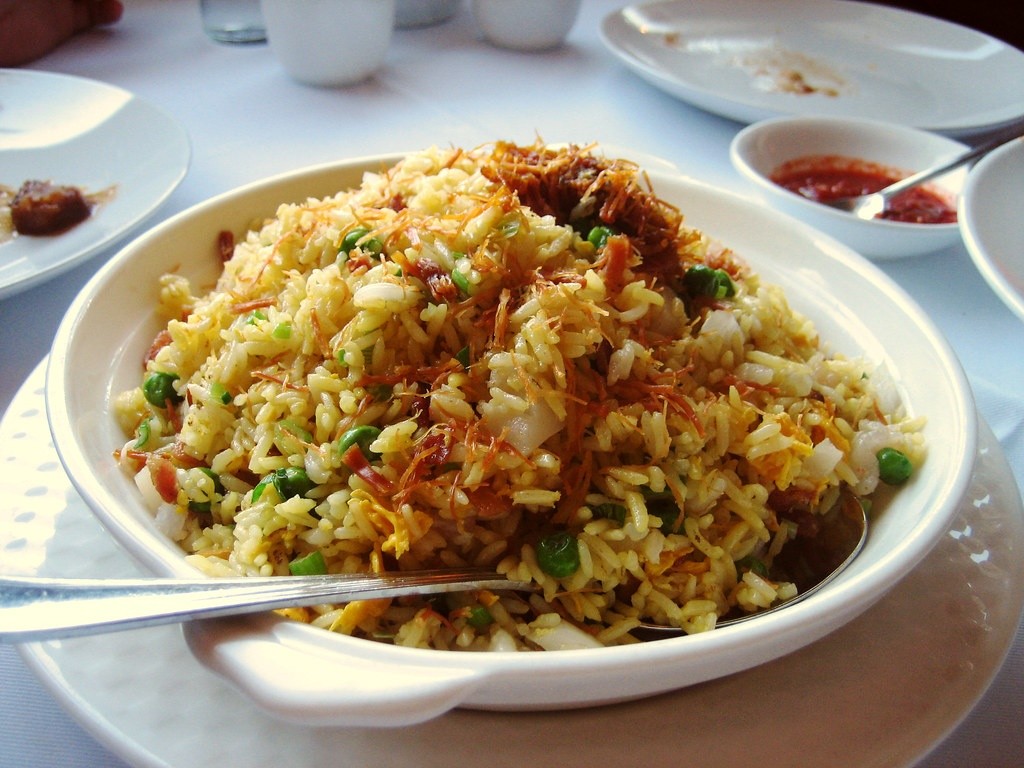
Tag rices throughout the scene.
[115,141,925,647]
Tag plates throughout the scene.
[596,1,1024,138]
[2,69,193,303]
[2,349,1023,767]
[962,131,1024,325]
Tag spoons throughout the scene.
[2,485,877,632]
[825,122,1024,222]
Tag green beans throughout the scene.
[144,223,912,625]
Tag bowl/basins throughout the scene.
[728,118,978,263]
[44,144,977,728]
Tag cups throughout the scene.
[259,0,394,89]
[198,1,268,43]
[395,1,457,29]
[472,0,582,51]
